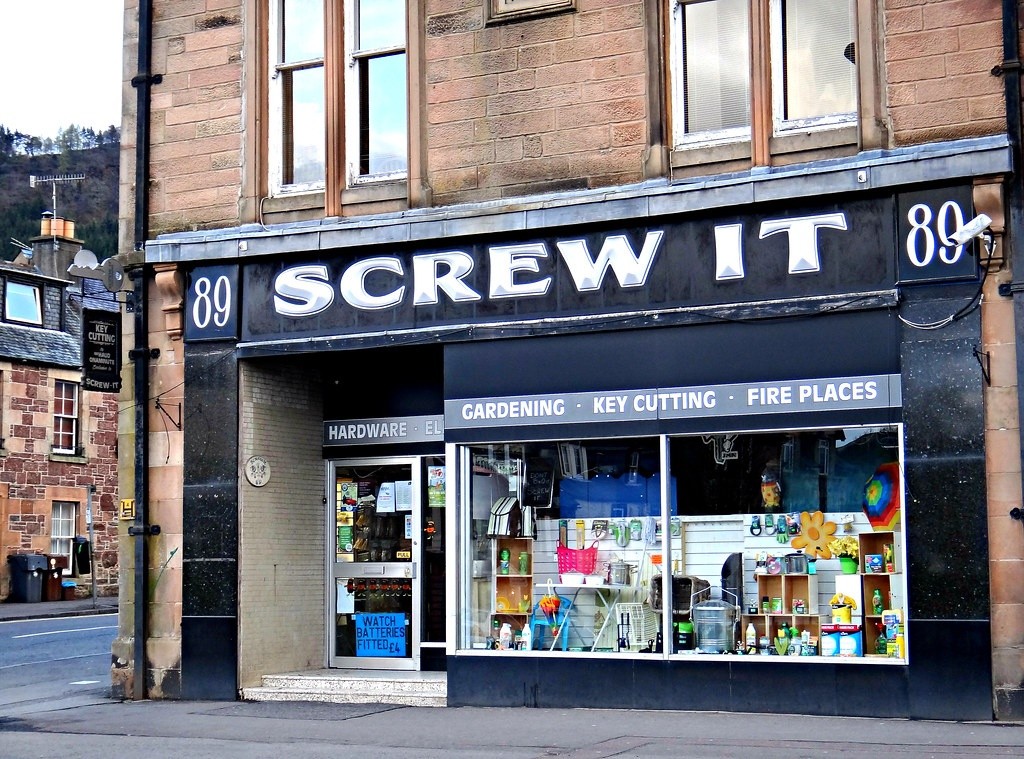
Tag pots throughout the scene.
[558,567,606,586]
[603,559,638,586]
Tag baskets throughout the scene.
[556,539,599,584]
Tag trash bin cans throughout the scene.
[37,553,68,601]
[7,554,48,604]
[62,582,77,600]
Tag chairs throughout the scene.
[531,596,576,652]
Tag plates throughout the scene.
[569,648,582,651]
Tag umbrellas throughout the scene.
[862,461,900,532]
[539,578,561,640]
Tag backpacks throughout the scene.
[518,504,537,540]
[486,498,521,537]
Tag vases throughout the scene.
[839,558,858,575]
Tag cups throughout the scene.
[784,555,807,574]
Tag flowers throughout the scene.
[790,511,837,560]
[828,536,859,557]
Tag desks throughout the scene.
[537,583,641,652]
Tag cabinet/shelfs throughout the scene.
[738,573,823,656]
[858,532,905,658]
[489,538,532,649]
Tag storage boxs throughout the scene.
[649,572,710,615]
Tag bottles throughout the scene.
[499,622,512,647]
[746,623,756,654]
[896,634,905,658]
[522,624,532,650]
